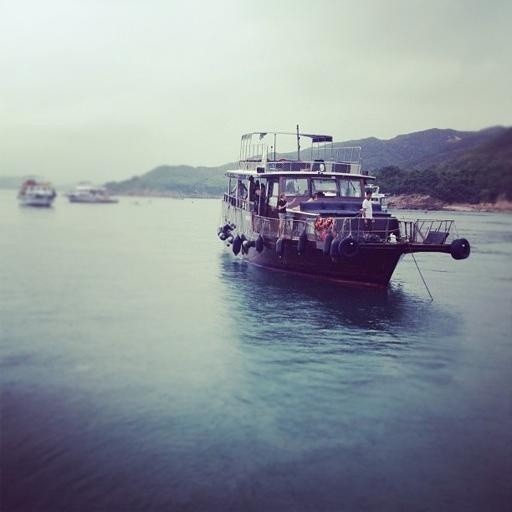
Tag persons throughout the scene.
[275,193,296,238]
[362,189,373,234]
[231,180,266,212]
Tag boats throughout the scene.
[17,179,56,207]
[66,182,121,205]
[218,124,471,290]
